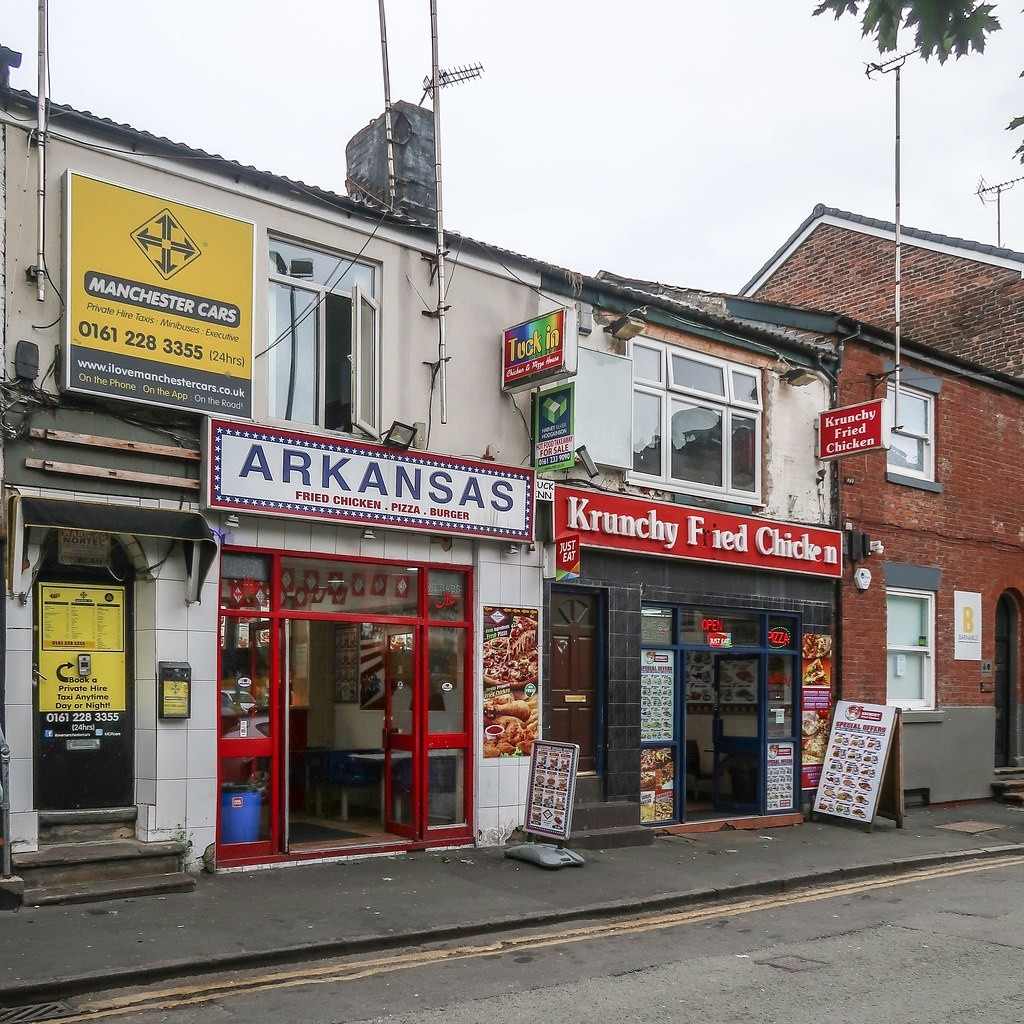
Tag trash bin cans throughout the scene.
[221,782,266,844]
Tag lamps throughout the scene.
[505,543,520,554]
[779,368,819,386]
[361,526,376,539]
[224,511,242,529]
[379,420,418,450]
[602,315,647,344]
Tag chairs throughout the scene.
[685,739,716,802]
[307,749,456,822]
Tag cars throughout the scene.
[221,690,256,714]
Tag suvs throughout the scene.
[220,713,271,803]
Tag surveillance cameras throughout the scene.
[875,545,884,555]
[576,445,600,479]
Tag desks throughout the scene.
[348,749,460,825]
[290,747,386,821]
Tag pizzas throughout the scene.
[508,616,538,653]
[482,637,538,687]
